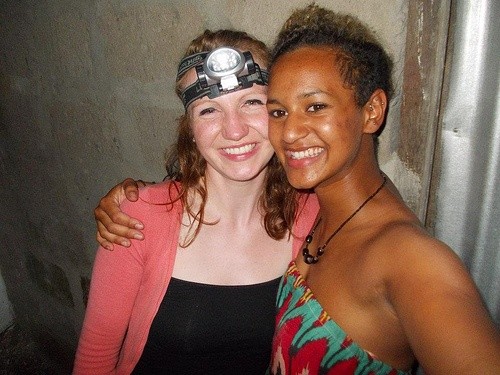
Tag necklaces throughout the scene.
[302,172,390,264]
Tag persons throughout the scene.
[93,5,500,375]
[70,29,322,374]
